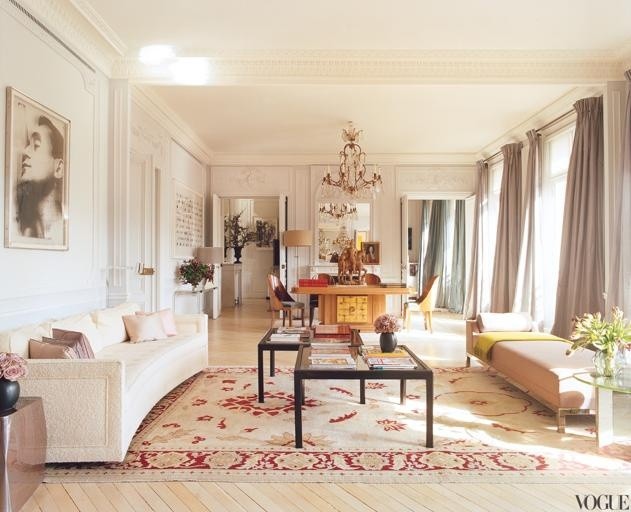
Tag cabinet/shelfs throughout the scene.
[0,396,47,512]
[221,263,244,307]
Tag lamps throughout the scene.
[319,202,357,221]
[321,121,383,196]
[196,247,223,263]
[284,229,313,320]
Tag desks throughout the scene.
[573,370,630,450]
[293,345,433,448]
[257,328,365,404]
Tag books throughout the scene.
[270,324,419,371]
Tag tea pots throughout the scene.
[351,330,364,346]
[348,346,362,361]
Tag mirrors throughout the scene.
[313,201,373,265]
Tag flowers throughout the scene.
[175,257,213,286]
[565,306,631,378]
[0,351,28,381]
[375,314,399,334]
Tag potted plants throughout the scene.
[224,209,253,263]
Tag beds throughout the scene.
[465,313,598,433]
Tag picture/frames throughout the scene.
[4,86,71,251]
[361,242,380,265]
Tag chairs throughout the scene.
[267,274,440,334]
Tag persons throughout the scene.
[15,115,65,239]
[363,245,378,263]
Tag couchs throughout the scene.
[0,302,208,463]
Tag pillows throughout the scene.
[477,313,532,331]
[29,307,177,360]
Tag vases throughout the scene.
[0,378,20,412]
[380,332,397,353]
[592,350,623,377]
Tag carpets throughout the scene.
[42,367,631,483]
